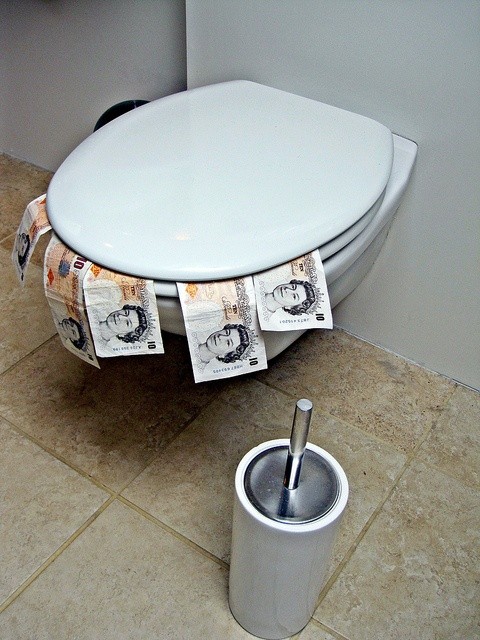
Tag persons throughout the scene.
[89,303,154,351]
[55,313,91,352]
[191,323,261,373]
[11,232,33,272]
[258,276,323,322]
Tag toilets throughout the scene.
[44,80,419,362]
